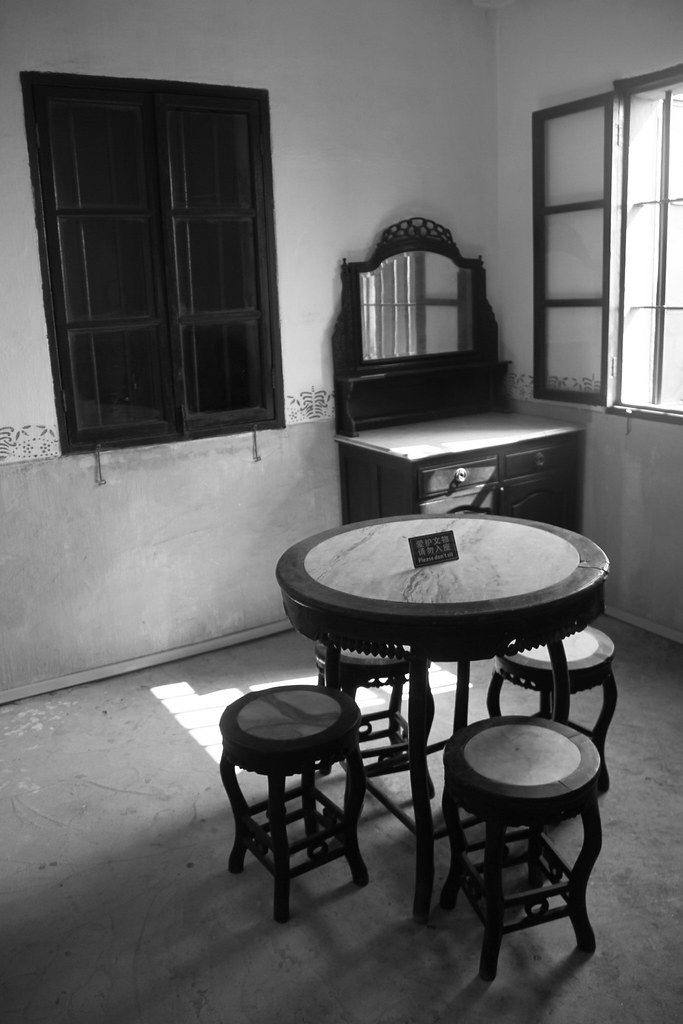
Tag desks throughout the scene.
[276,514,610,923]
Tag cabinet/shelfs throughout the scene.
[335,430,584,527]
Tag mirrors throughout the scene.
[331,216,513,437]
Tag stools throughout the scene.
[218,685,368,923]
[315,641,409,776]
[440,715,608,980]
[487,622,619,791]
